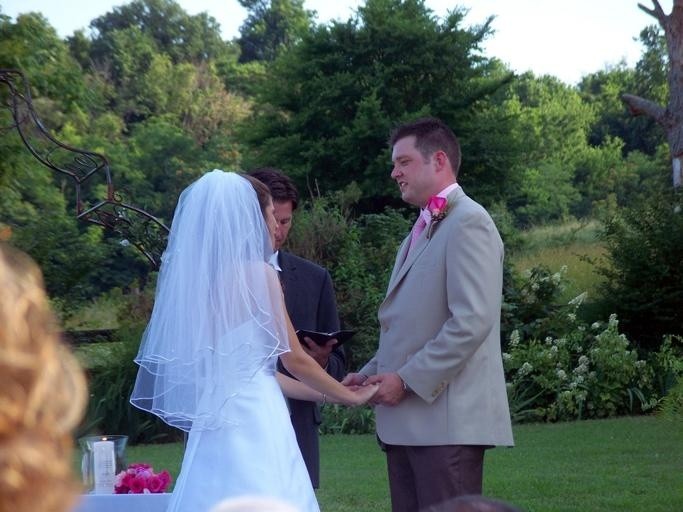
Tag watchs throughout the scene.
[401,380,413,392]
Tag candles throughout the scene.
[93,436,114,493]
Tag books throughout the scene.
[295,327,357,353]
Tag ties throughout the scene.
[409,214,427,249]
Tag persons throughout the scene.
[0,225,90,510]
[248,164,346,511]
[126,164,385,512]
[337,116,516,511]
[422,491,520,510]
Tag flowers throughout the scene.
[112,463,171,494]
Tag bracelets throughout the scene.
[317,392,328,410]
